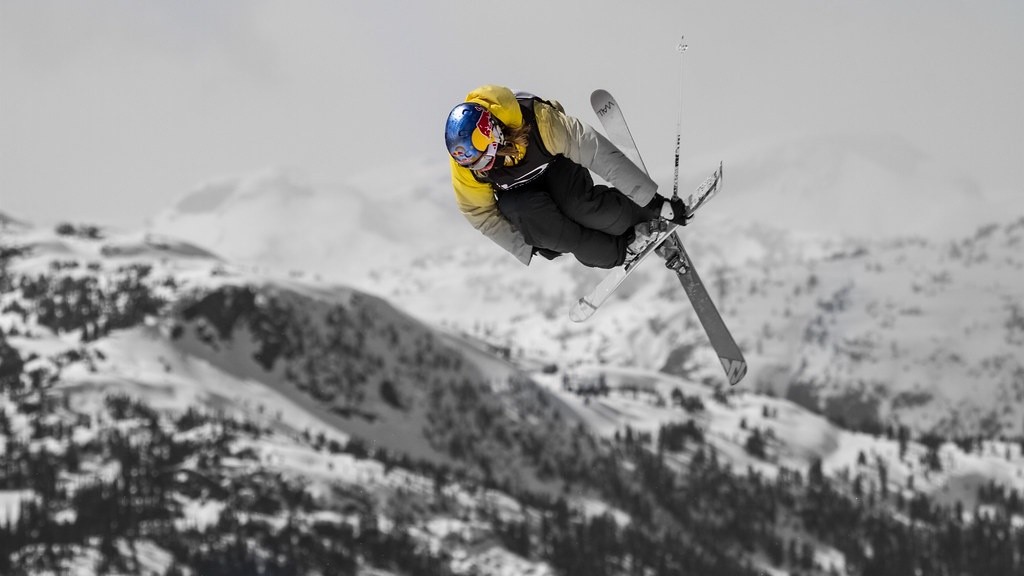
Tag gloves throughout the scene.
[643,193,686,227]
[532,247,561,260]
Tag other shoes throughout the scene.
[620,198,651,255]
[614,236,638,267]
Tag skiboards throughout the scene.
[567,89,748,386]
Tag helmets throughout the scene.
[444,102,495,165]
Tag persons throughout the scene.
[445,85,695,270]
